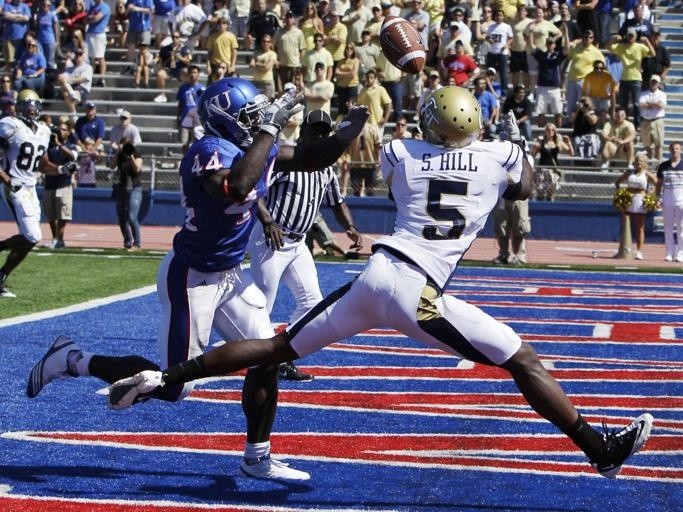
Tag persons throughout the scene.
[26,78,370,482]
[0,89,80,297]
[106,86,653,480]
[244,110,362,381]
[1,0,681,265]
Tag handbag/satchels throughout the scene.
[555,169,563,181]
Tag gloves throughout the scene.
[63,162,76,175]
[500,109,521,142]
[259,89,305,144]
[334,105,370,145]
[8,181,21,191]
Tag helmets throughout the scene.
[17,89,42,126]
[420,86,481,148]
[197,77,271,151]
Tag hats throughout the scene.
[120,110,130,119]
[86,102,95,108]
[455,40,464,46]
[372,5,381,9]
[488,67,496,73]
[449,21,459,28]
[304,110,332,131]
[285,10,294,16]
[626,26,636,35]
[546,37,554,42]
[650,73,661,83]
[428,70,438,77]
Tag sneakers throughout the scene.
[0,283,16,297]
[279,362,313,382]
[240,457,311,481]
[94,79,106,87]
[635,251,642,259]
[494,255,508,265]
[110,370,169,409]
[589,413,653,478]
[28,335,81,397]
[513,256,526,265]
[665,255,674,261]
[45,239,64,249]
[127,245,142,251]
[675,255,683,262]
[154,95,167,103]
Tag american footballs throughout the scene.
[382,15,425,74]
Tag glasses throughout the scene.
[483,10,492,13]
[454,13,462,17]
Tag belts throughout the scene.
[283,231,303,244]
